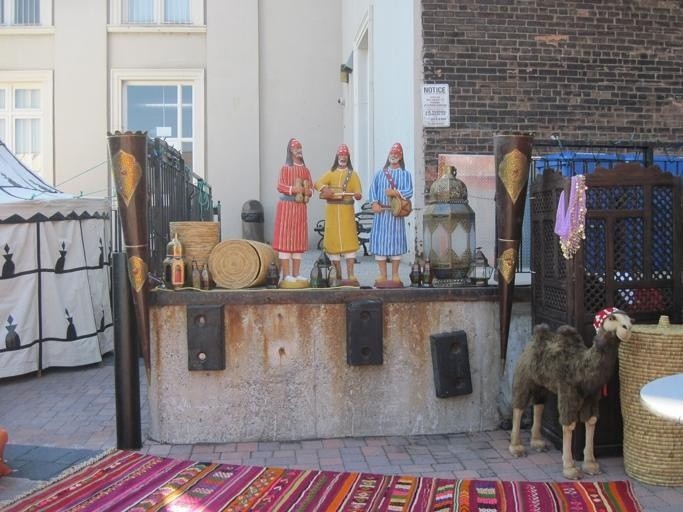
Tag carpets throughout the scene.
[1,447,644,511]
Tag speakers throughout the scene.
[187,305,224,371]
[429,330,473,398]
[346,302,383,366]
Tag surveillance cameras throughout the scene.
[336,96,345,107]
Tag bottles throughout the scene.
[190,258,203,290]
[202,262,214,291]
[162,241,186,289]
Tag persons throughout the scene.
[368,142,414,284]
[311,143,364,283]
[275,137,316,283]
